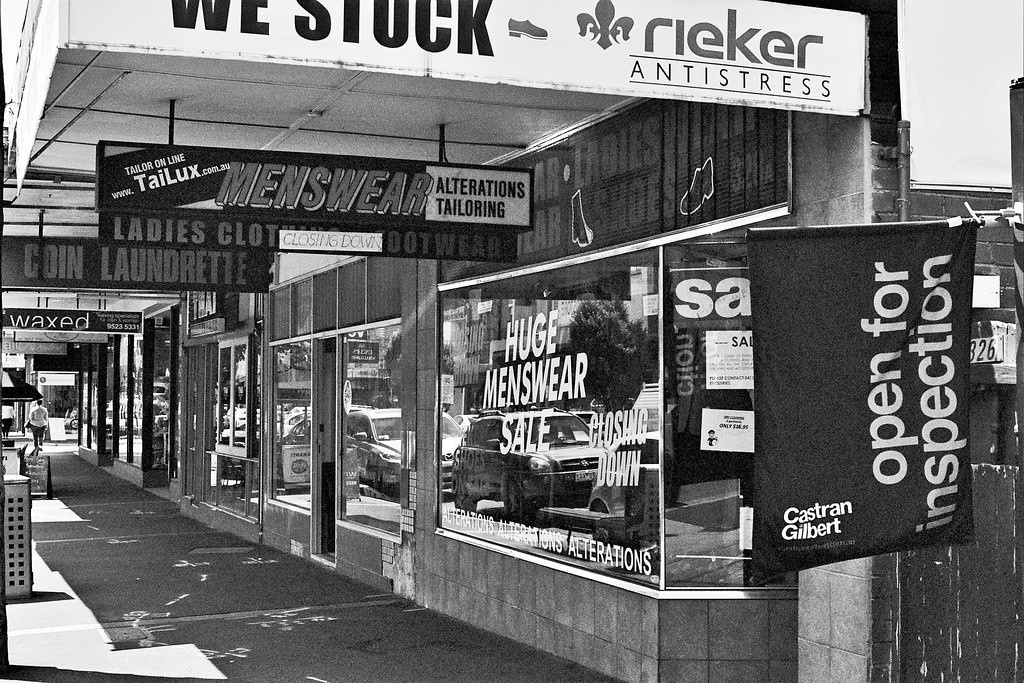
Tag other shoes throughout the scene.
[35,447,38,456]
[39,447,42,452]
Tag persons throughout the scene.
[29,400,48,451]
[2,402,15,439]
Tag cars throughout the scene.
[349,405,374,413]
[588,431,659,547]
[453,415,479,434]
[227,408,260,429]
[276,411,311,440]
[288,407,311,414]
[281,418,311,445]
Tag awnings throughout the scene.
[2,371,43,402]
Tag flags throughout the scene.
[744,224,977,574]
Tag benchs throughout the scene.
[539,507,619,547]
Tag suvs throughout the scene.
[453,406,611,523]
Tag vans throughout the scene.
[346,408,465,505]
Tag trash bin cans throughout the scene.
[624,464,660,568]
[3,475,34,604]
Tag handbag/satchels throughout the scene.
[24,418,31,429]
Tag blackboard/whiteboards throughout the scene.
[22,454,51,501]
